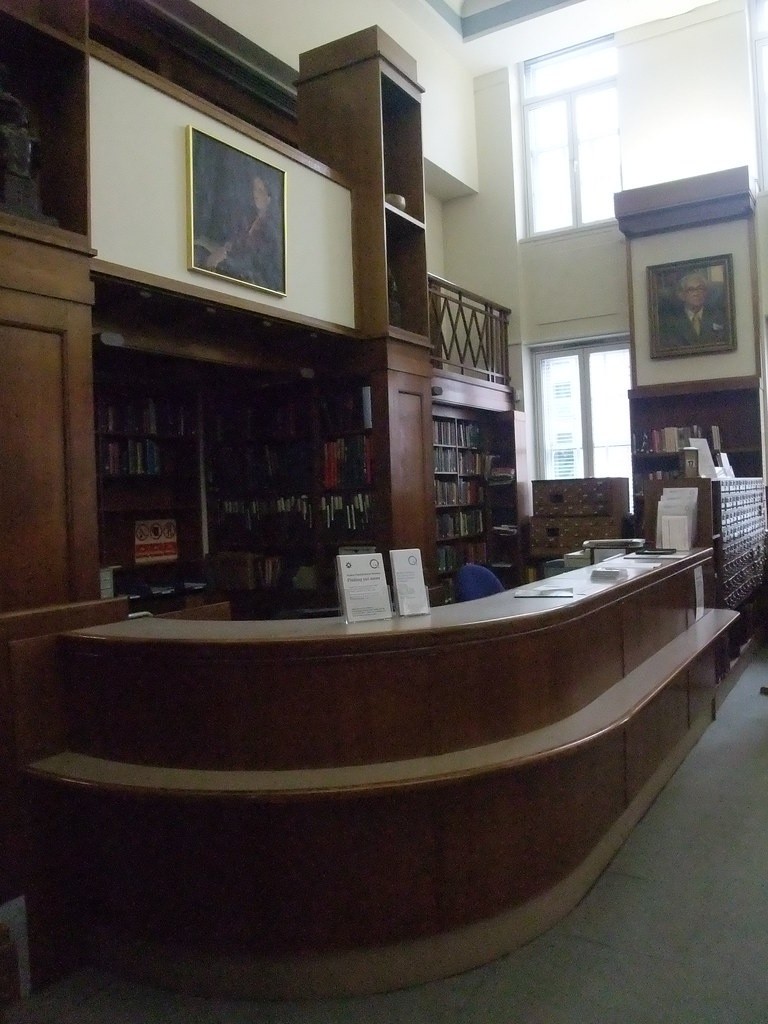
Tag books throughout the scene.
[435,509,520,538]
[513,586,574,598]
[431,419,481,447]
[96,433,376,489]
[631,422,723,455]
[436,542,489,571]
[433,478,490,505]
[433,448,516,479]
[634,469,683,496]
[211,491,377,544]
[257,556,282,583]
[92,386,366,434]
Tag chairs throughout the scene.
[456,565,505,603]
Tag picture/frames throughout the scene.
[645,254,738,360]
[185,125,288,298]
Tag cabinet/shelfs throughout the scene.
[0,0,768,715]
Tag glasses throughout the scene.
[686,286,704,293]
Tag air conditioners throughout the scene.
[613,166,756,238]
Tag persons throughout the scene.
[213,172,285,293]
[657,272,730,352]
